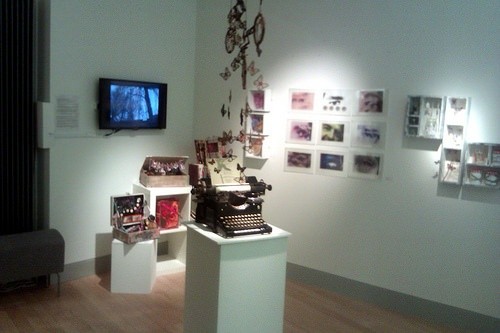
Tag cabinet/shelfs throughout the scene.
[438,96,471,186]
[404,94,445,140]
[133,184,192,272]
[244,88,268,160]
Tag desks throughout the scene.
[110,238,159,294]
[183,220,290,333]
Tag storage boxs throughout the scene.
[110,193,161,244]
[140,155,191,187]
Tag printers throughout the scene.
[191,175,273,238]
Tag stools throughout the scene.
[0,229,64,297]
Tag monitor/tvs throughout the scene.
[98,78,168,129]
